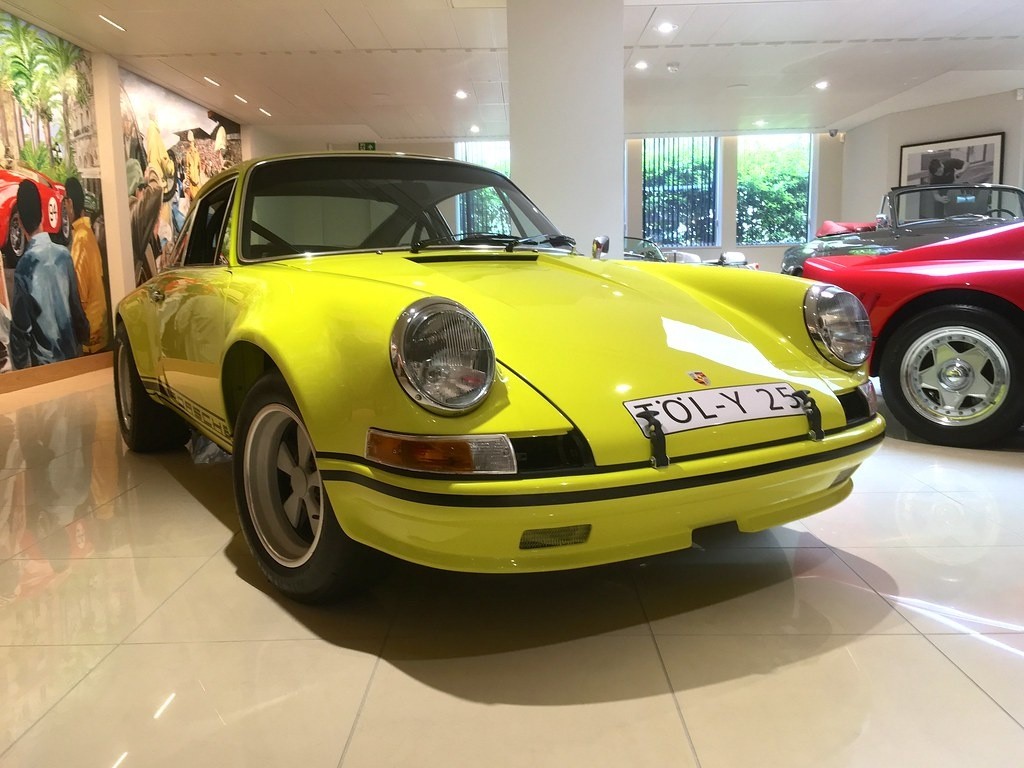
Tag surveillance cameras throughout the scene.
[829,129,838,137]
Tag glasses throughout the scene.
[137,184,147,191]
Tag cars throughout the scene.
[780,182,1024,447]
[112,149,886,605]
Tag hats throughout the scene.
[188,130,194,141]
[126,158,145,198]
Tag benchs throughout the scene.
[815,219,876,237]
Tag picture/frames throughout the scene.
[896,131,1006,225]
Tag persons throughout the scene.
[929,158,969,219]
